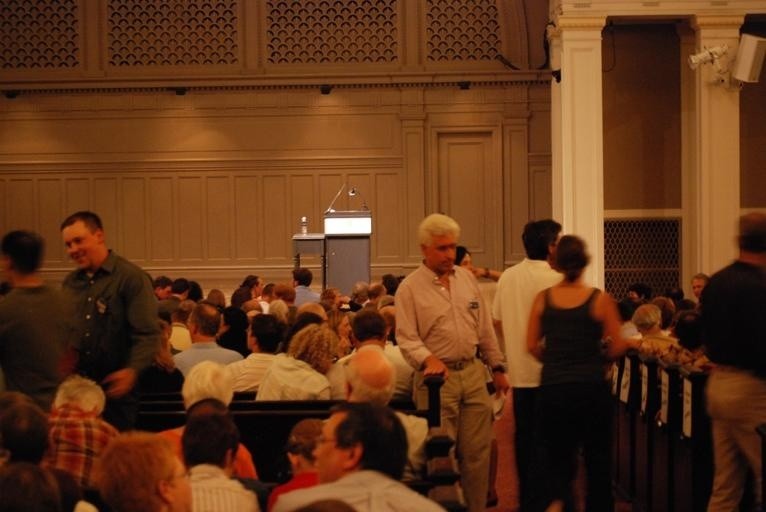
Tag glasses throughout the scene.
[311,432,343,446]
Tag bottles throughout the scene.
[301,216,308,237]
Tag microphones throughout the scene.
[349,187,368,211]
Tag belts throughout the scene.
[441,357,474,371]
[710,362,749,375]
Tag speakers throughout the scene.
[733,34,765,83]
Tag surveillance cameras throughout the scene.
[687,42,729,71]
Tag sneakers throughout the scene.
[486,491,499,508]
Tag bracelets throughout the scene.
[492,364,506,373]
[485,267,489,278]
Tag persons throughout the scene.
[0,229,79,406]
[525,235,628,510]
[700,211,766,512]
[493,220,565,512]
[395,214,509,511]
[59,210,157,430]
[453,242,508,506]
[611,270,714,435]
[0,266,448,512]
